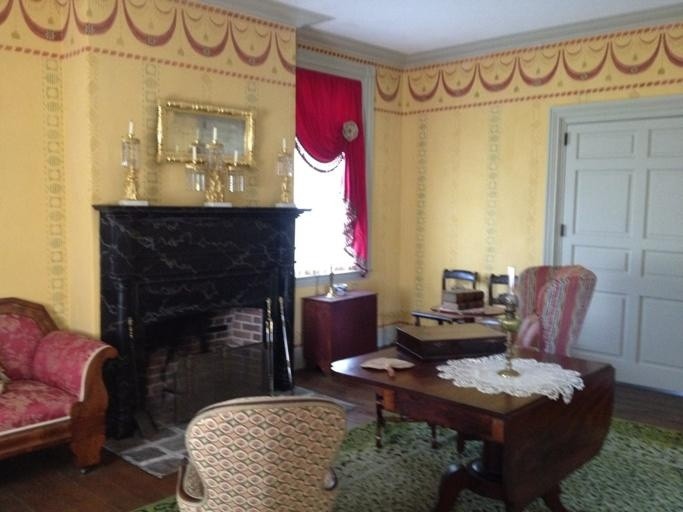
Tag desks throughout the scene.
[430,303,514,324]
[327,338,616,510]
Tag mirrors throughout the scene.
[153,99,257,171]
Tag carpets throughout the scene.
[102,380,354,484]
[129,411,682,511]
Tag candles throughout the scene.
[126,118,136,138]
[506,264,516,295]
[281,136,287,153]
[212,126,218,144]
[190,144,198,162]
[233,150,238,166]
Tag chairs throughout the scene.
[408,269,478,326]
[0,295,117,471]
[174,395,352,511]
[455,262,598,454]
[486,273,518,308]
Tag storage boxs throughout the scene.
[441,288,485,310]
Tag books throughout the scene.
[442,285,484,302]
[440,298,482,310]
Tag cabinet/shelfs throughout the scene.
[299,287,378,378]
